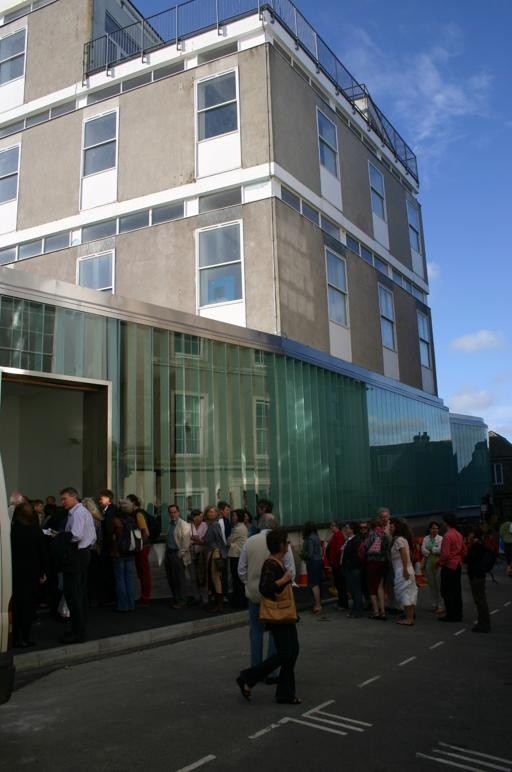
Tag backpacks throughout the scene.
[134,508,162,545]
[471,542,497,573]
[118,519,143,558]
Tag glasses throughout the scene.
[359,525,368,529]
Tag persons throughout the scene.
[296,506,511,634]
[10,486,280,643]
[236,512,302,703]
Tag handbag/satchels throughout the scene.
[257,556,298,624]
[203,547,222,560]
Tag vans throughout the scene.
[0,455,20,707]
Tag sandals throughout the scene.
[275,693,303,705]
[234,673,253,702]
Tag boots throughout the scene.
[207,593,225,613]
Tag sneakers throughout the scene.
[171,600,189,609]
[261,676,279,685]
[56,630,87,644]
[311,602,491,633]
[11,638,37,649]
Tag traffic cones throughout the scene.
[297,560,309,588]
[413,549,429,587]
